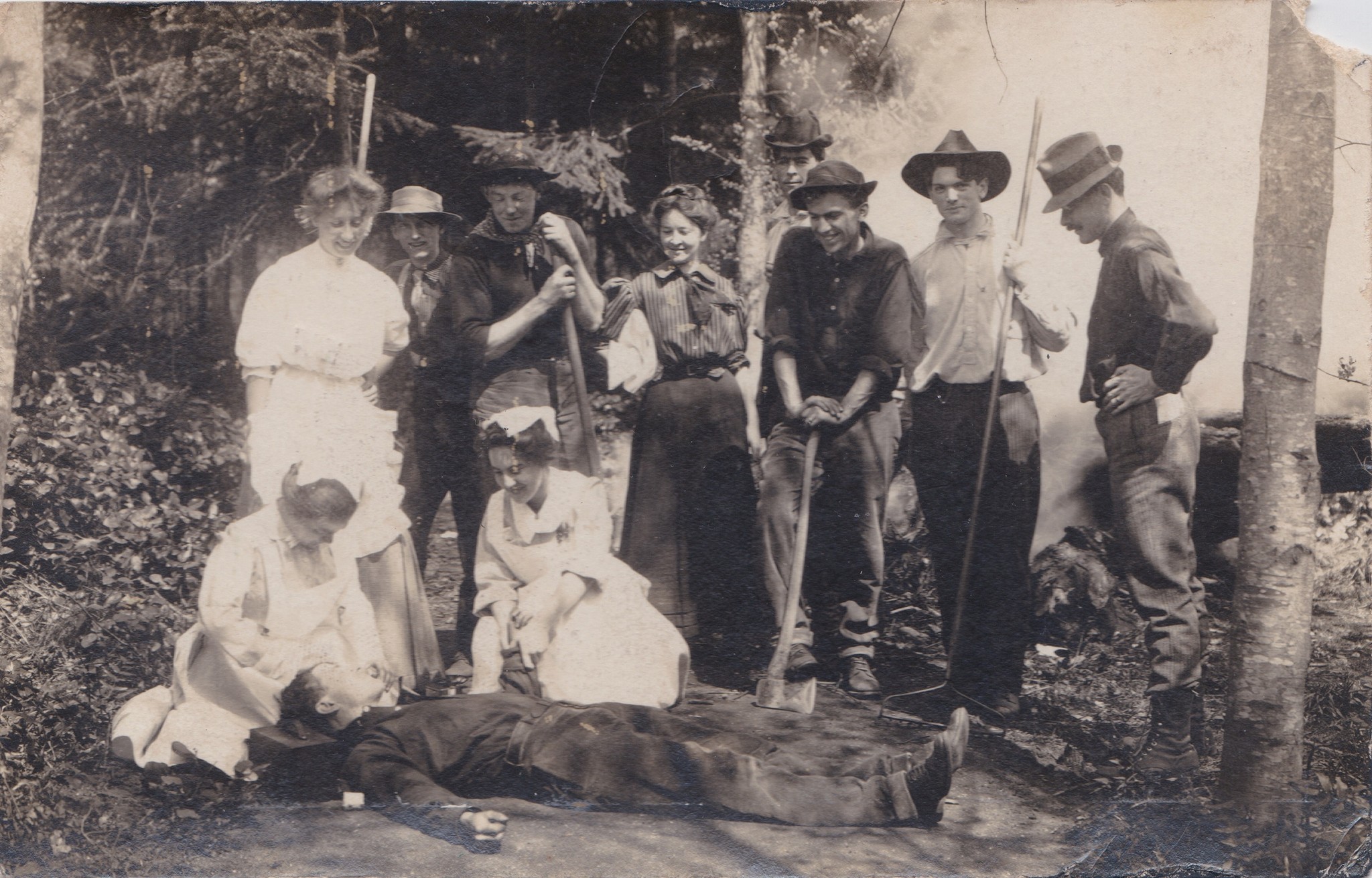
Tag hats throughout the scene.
[460,148,562,189]
[902,130,1011,202]
[764,109,834,148]
[791,160,880,211]
[1036,131,1121,214]
[376,186,463,224]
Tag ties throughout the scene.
[411,268,434,333]
[683,273,738,328]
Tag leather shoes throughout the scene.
[846,654,884,696]
[785,644,819,675]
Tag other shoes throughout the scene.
[992,689,1019,715]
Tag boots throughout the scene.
[1097,686,1217,776]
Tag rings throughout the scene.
[1110,397,1121,406]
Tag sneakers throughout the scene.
[908,707,969,774]
[904,738,952,822]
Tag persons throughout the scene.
[282,658,970,859]
[106,458,401,781]
[466,406,693,715]
[599,181,779,651]
[442,158,607,518]
[1036,129,1221,776]
[231,167,442,697]
[888,128,1075,725]
[744,105,834,509]
[751,160,915,698]
[378,184,505,667]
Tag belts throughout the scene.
[507,698,554,796]
